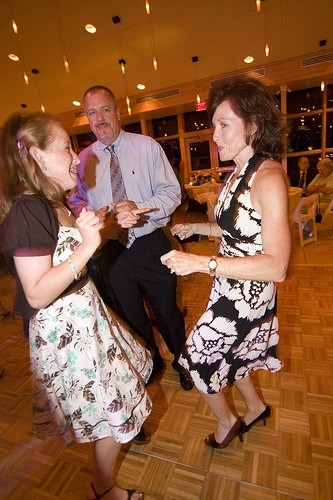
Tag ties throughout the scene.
[300,172,305,188]
[105,144,136,249]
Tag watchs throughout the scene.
[208,256,218,276]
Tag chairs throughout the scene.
[291,194,333,247]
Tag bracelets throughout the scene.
[207,221,212,238]
[68,254,80,281]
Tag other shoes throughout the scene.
[147,355,164,386]
[172,360,194,391]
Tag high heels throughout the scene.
[204,417,246,448]
[240,404,271,433]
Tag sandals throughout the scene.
[90,482,144,500]
[133,429,151,444]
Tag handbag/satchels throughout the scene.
[315,214,322,223]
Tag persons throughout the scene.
[0,112,153,500]
[67,86,198,391]
[160,76,333,450]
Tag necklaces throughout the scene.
[59,204,72,216]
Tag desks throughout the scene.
[184,182,219,205]
[204,185,304,241]
